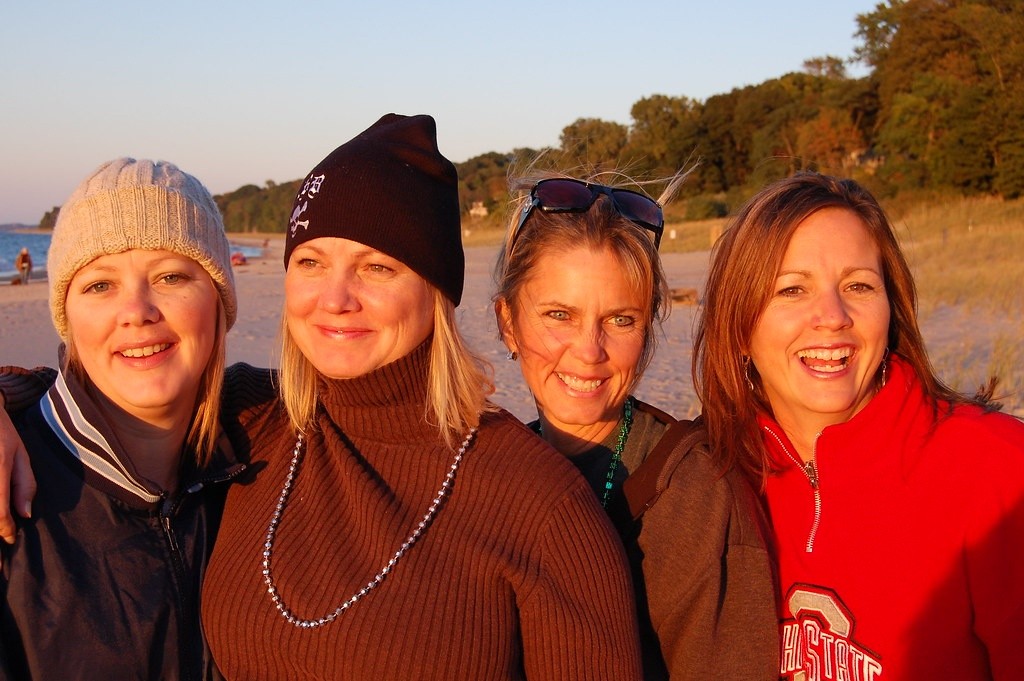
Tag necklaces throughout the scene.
[538,397,633,511]
[263,431,476,628]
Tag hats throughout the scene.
[47,157,237,346]
[284,113,465,307]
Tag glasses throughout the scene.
[510,178,664,255]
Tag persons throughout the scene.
[0,157,238,681]
[491,167,781,681]
[15,247,32,285]
[692,171,1024,681]
[0,174,634,681]
